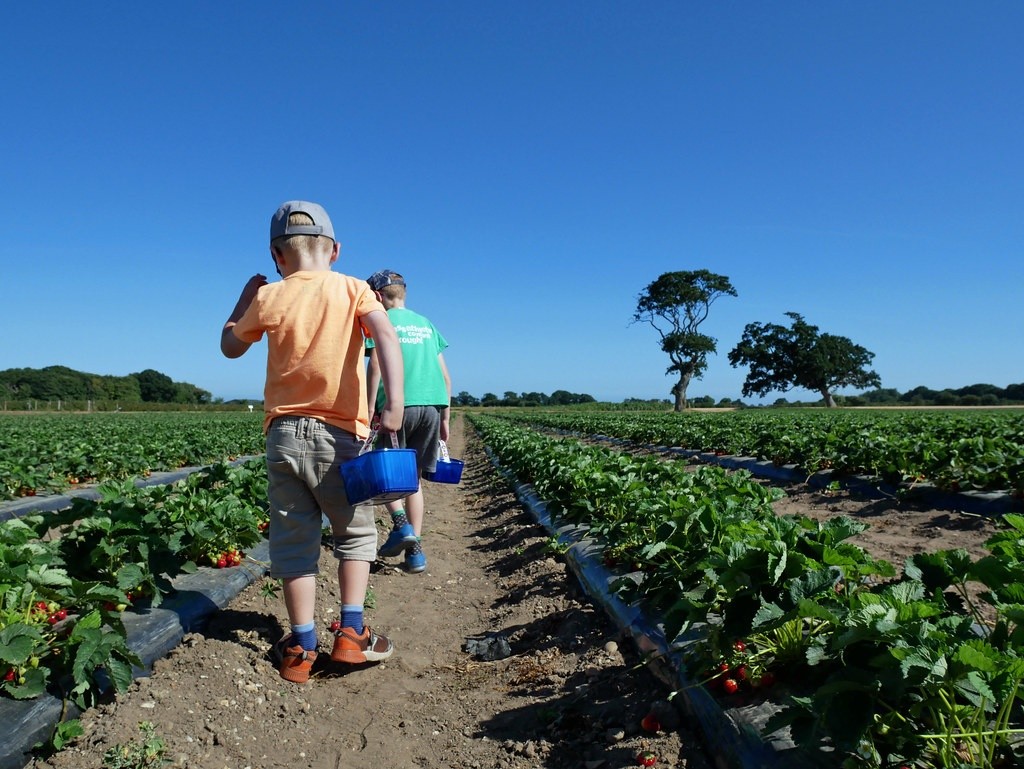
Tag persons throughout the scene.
[364,269,451,573]
[219,199,407,682]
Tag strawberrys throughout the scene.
[639,628,775,766]
[635,562,642,569]
[5,516,342,685]
[21,470,152,498]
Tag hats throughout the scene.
[366,269,406,291]
[270,199,336,276]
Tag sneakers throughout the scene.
[276,631,320,682]
[331,625,394,664]
[378,525,417,557]
[405,552,426,572]
